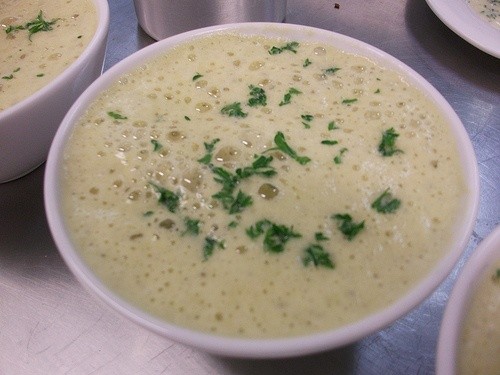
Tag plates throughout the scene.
[425,0,500,62]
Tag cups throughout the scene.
[133,0,288,42]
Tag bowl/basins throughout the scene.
[44,23,480,356]
[436,221,500,375]
[0,0,111,186]
